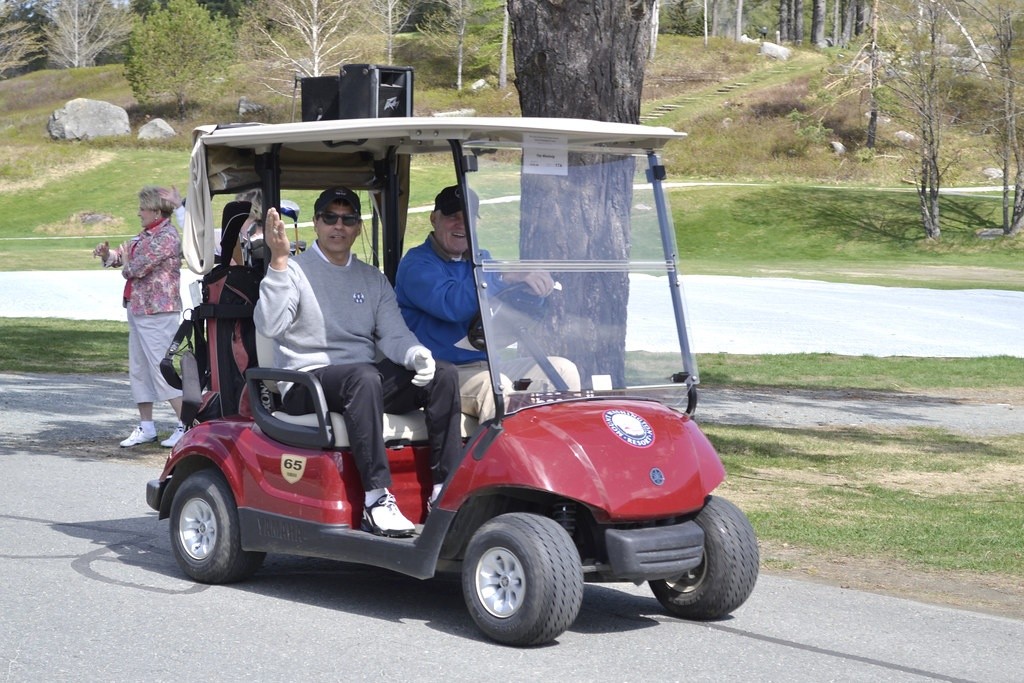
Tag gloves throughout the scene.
[406,345,437,387]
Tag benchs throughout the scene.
[235,288,490,446]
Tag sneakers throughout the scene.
[427,496,436,516]
[361,494,417,539]
[160,428,190,448]
[118,426,158,447]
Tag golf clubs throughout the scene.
[278,198,303,257]
[237,220,258,275]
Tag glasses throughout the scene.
[319,211,360,226]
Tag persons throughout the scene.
[94,187,191,447]
[159,186,264,267]
[253,186,463,538]
[393,183,581,427]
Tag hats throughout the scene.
[313,186,363,213]
[433,185,482,220]
